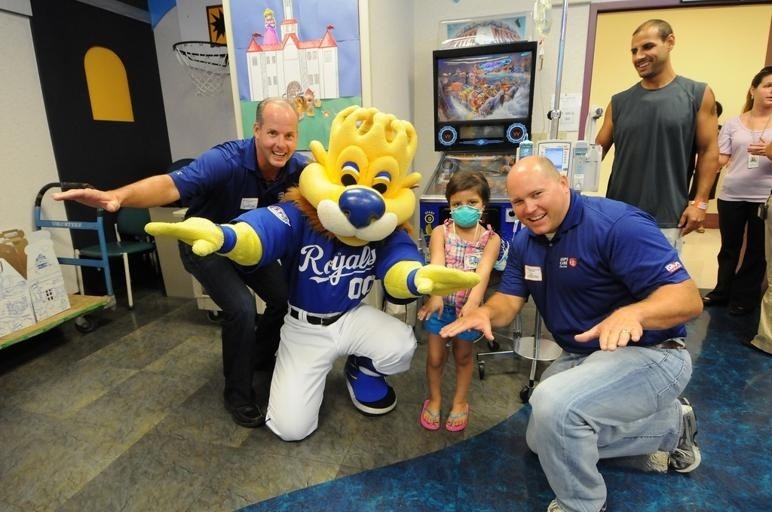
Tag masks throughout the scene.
[450,205,484,228]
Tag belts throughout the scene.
[656,339,685,349]
[290,308,347,325]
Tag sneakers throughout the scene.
[667,397,702,473]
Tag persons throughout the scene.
[437,153,705,512]
[416,169,503,433]
[592,17,721,254]
[48,97,420,430]
[687,99,724,201]
[742,136,771,357]
[700,65,771,314]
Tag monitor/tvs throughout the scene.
[432,41,538,152]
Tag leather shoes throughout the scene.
[702,292,725,304]
[730,306,746,316]
[226,400,264,427]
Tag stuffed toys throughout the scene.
[144,102,483,444]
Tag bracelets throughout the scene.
[689,197,710,211]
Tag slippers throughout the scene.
[445,403,469,432]
[420,399,440,430]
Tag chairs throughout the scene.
[73,207,167,311]
